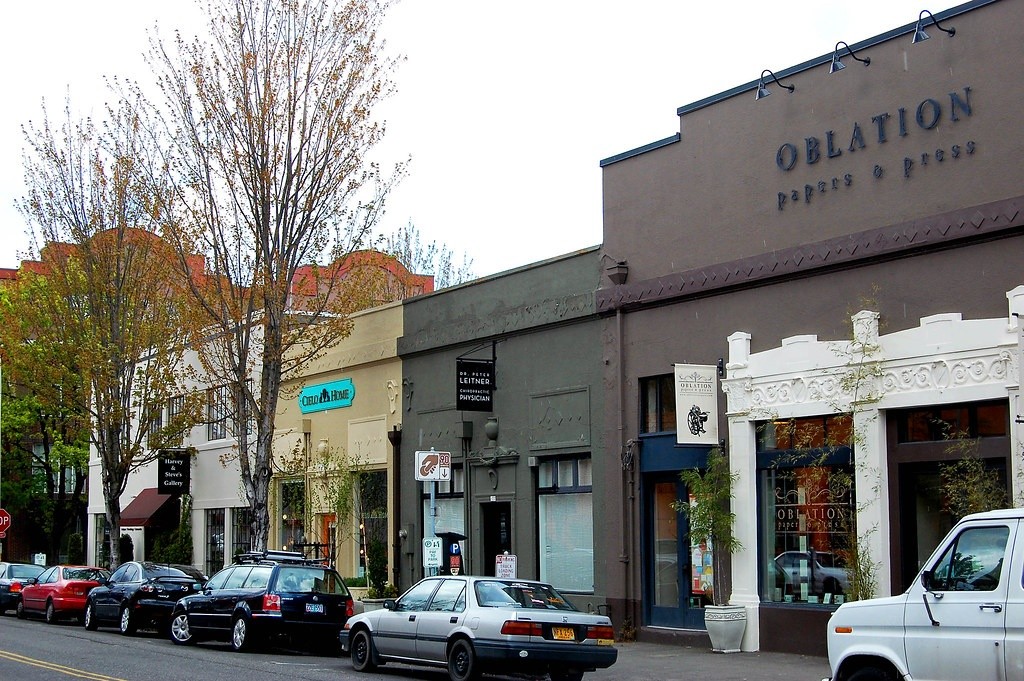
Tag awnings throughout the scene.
[120,488,172,526]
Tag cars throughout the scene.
[83,561,208,637]
[656,540,677,565]
[0,562,55,616]
[769,551,852,599]
[826,508,1024,681]
[16,565,112,625]
[340,576,619,681]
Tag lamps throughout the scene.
[912,10,955,44]
[756,70,795,101]
[829,41,870,74]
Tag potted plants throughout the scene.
[335,453,399,611]
[672,447,747,652]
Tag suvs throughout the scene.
[168,549,355,653]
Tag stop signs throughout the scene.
[0,509,11,533]
